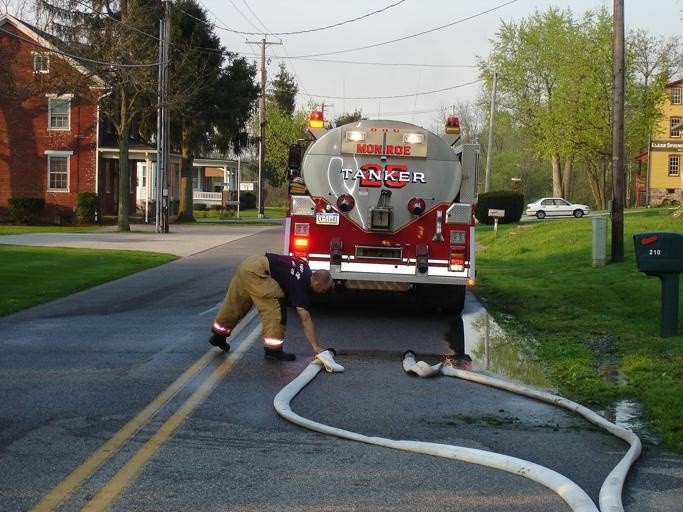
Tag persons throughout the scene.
[207,252,333,360]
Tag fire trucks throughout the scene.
[282,104,480,316]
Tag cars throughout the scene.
[526,197,592,219]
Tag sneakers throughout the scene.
[209,334,230,351]
[265,350,296,361]
